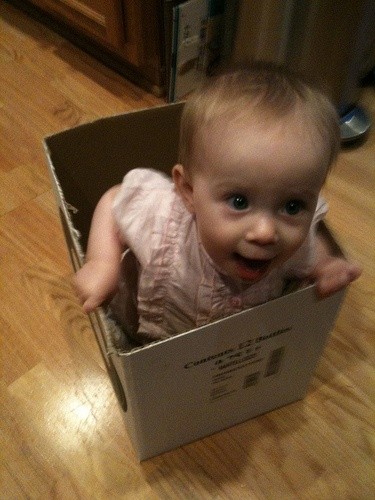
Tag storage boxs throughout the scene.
[41,99,360,463]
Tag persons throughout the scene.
[74,60,364,345]
